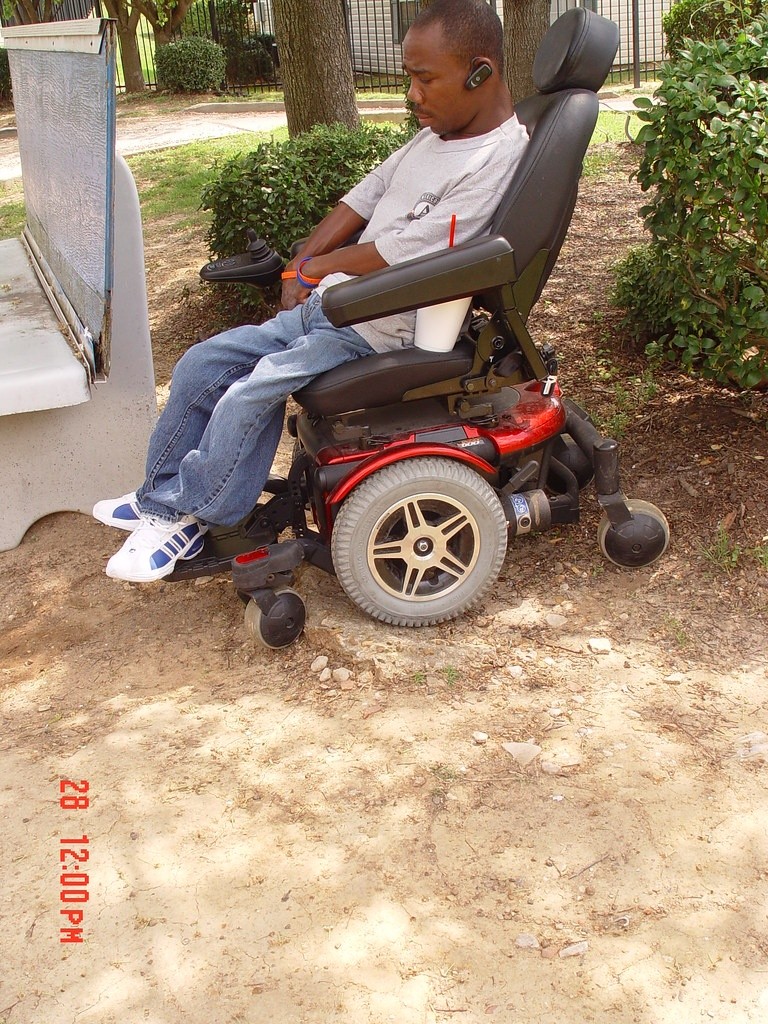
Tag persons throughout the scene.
[93,0,532,584]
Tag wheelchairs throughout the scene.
[156,8,669,653]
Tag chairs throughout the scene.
[198,7,621,418]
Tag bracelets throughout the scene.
[297,257,321,288]
[281,271,297,279]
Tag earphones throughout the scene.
[464,64,492,91]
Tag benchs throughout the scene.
[0,18,161,553]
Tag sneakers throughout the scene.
[91,492,209,535]
[105,515,205,583]
